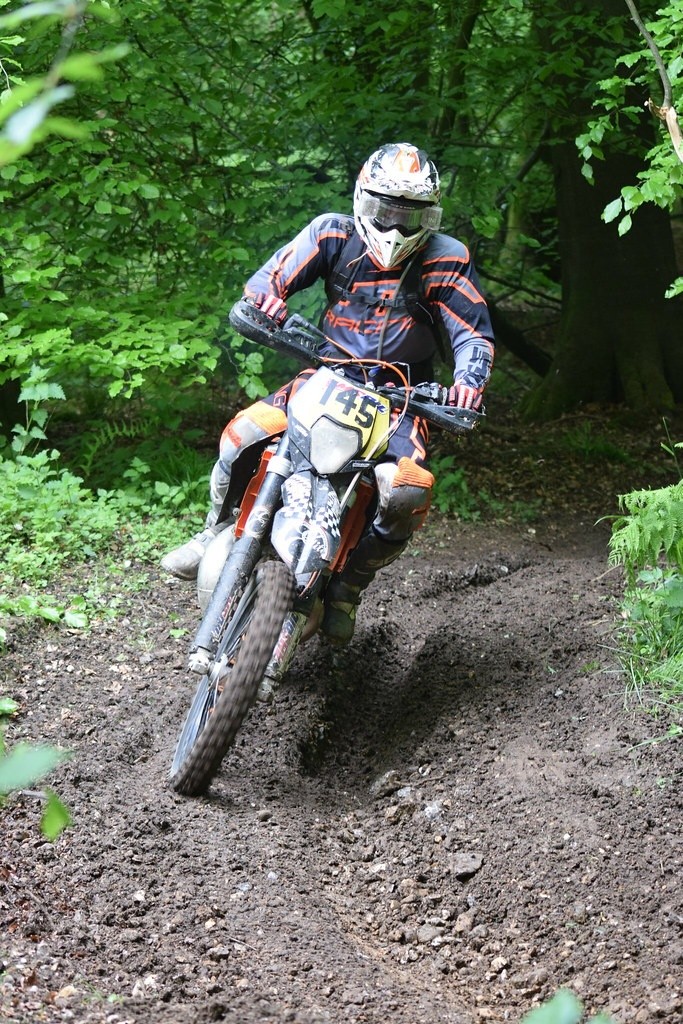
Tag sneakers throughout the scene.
[160,528,216,581]
[321,575,368,646]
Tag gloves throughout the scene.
[245,291,289,332]
[447,370,485,411]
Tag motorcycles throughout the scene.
[167,294,493,797]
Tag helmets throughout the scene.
[353,142,443,268]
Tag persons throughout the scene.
[158,143,494,650]
